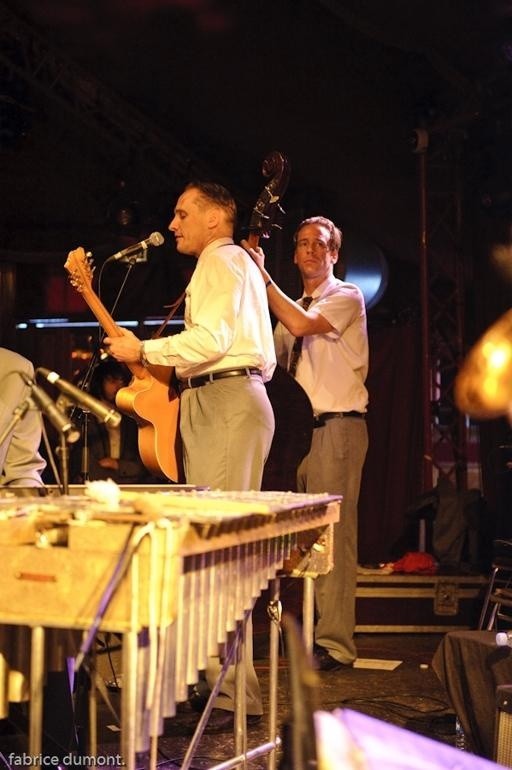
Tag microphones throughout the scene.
[18,371,81,444]
[37,367,122,428]
[105,231,165,262]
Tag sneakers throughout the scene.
[313,648,354,670]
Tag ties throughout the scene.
[288,296,314,378]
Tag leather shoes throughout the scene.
[189,707,262,734]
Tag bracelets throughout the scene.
[264,279,273,288]
[139,333,144,362]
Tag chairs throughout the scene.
[476,537,512,632]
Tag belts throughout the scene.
[179,365,261,390]
[314,409,362,429]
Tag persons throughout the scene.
[83,368,160,485]
[233,215,371,669]
[94,178,282,739]
[2,344,53,490]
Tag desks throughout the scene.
[430,629,512,770]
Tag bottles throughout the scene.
[455,715,471,752]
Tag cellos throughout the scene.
[247,151,314,492]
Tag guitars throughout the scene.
[65,247,184,484]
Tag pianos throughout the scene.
[0,483,342,767]
